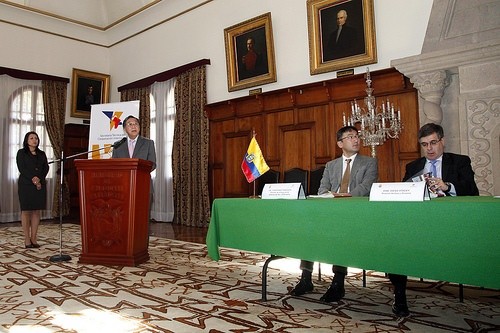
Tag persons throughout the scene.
[16,132,49,248]
[112,116,157,250]
[289,125,378,302]
[388,123,479,318]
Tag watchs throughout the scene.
[443,182,451,193]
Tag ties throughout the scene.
[128,139,135,158]
[339,159,352,193]
[430,160,437,180]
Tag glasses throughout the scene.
[420,137,443,147]
[127,122,139,127]
[339,135,361,141]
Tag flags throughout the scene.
[241,136,270,183]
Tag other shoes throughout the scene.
[31,240,39,247]
[25,240,33,248]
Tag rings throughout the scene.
[35,182,36,184]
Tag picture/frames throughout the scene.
[224,12,277,92]
[71,68,110,118]
[307,0,378,75]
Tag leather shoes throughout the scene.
[392,291,408,316]
[320,285,345,302]
[290,281,313,295]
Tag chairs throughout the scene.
[258,166,325,196]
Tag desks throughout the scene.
[206,196,500,302]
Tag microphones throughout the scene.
[114,136,127,149]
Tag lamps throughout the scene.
[343,67,402,158]
